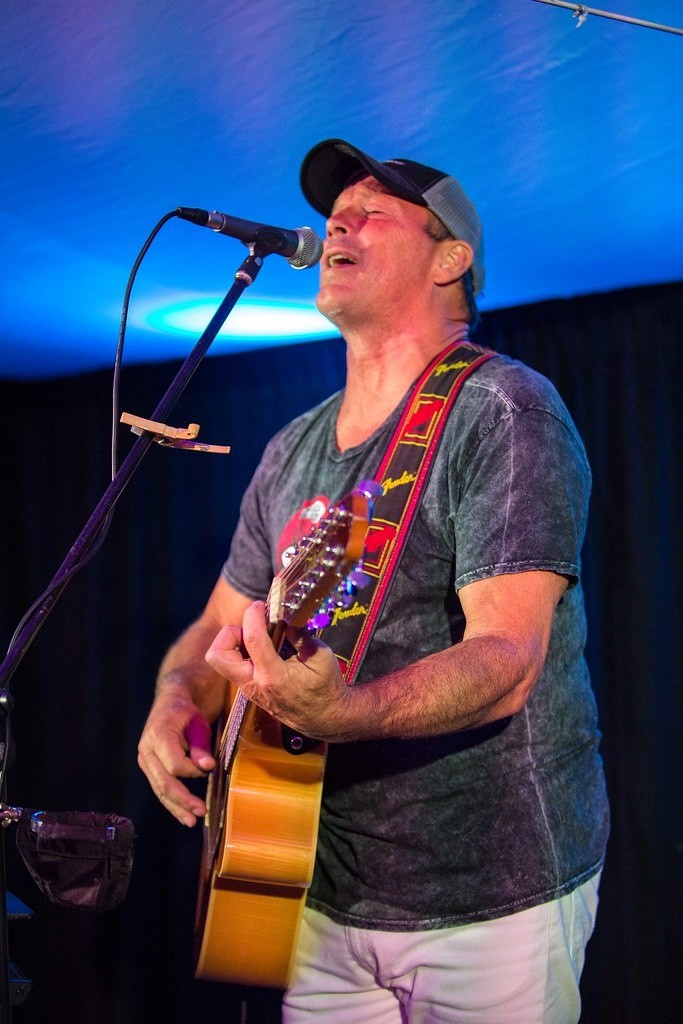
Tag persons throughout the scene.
[135,138,609,1023]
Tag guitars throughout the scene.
[197,493,370,985]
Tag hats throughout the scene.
[301,138,486,297]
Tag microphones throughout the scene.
[178,208,324,269]
